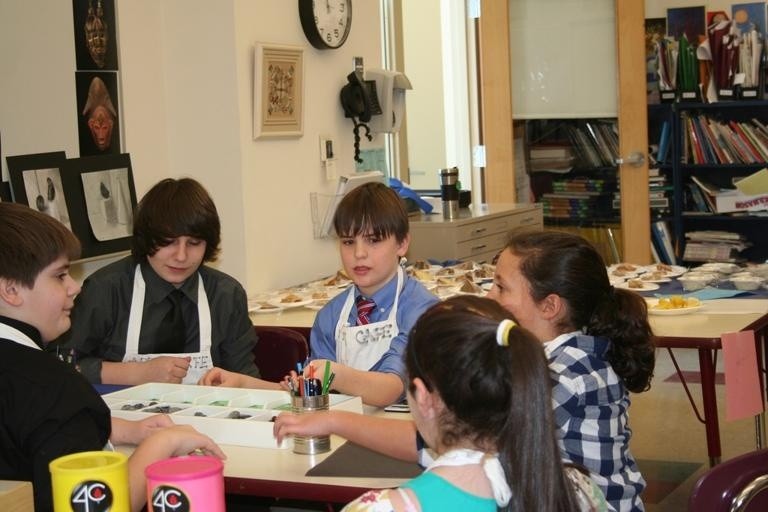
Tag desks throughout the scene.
[100,397,424,512]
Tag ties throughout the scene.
[352,296,374,326]
[150,291,190,354]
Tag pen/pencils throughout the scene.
[288,361,335,396]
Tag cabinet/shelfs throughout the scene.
[672,98,768,267]
[406,204,543,263]
[522,104,677,227]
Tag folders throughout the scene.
[319,170,385,237]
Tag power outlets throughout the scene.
[319,134,338,180]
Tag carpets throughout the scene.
[634,458,705,505]
[664,370,725,384]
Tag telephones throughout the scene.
[340,70,382,163]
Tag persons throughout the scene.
[48,177,258,388]
[194,181,443,412]
[338,292,615,511]
[270,230,658,512]
[0,197,227,512]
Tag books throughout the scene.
[534,175,676,221]
[681,167,768,218]
[543,222,678,269]
[678,110,767,165]
[680,229,755,265]
[524,116,673,177]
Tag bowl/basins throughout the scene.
[675,262,766,290]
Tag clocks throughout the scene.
[298,0,352,50]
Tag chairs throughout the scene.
[688,448,768,512]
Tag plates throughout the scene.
[646,296,703,315]
[249,256,494,318]
[606,262,685,291]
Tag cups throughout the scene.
[440,169,460,220]
[144,457,224,512]
[47,453,133,512]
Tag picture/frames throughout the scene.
[6,151,76,237]
[253,42,308,140]
[67,153,138,259]
